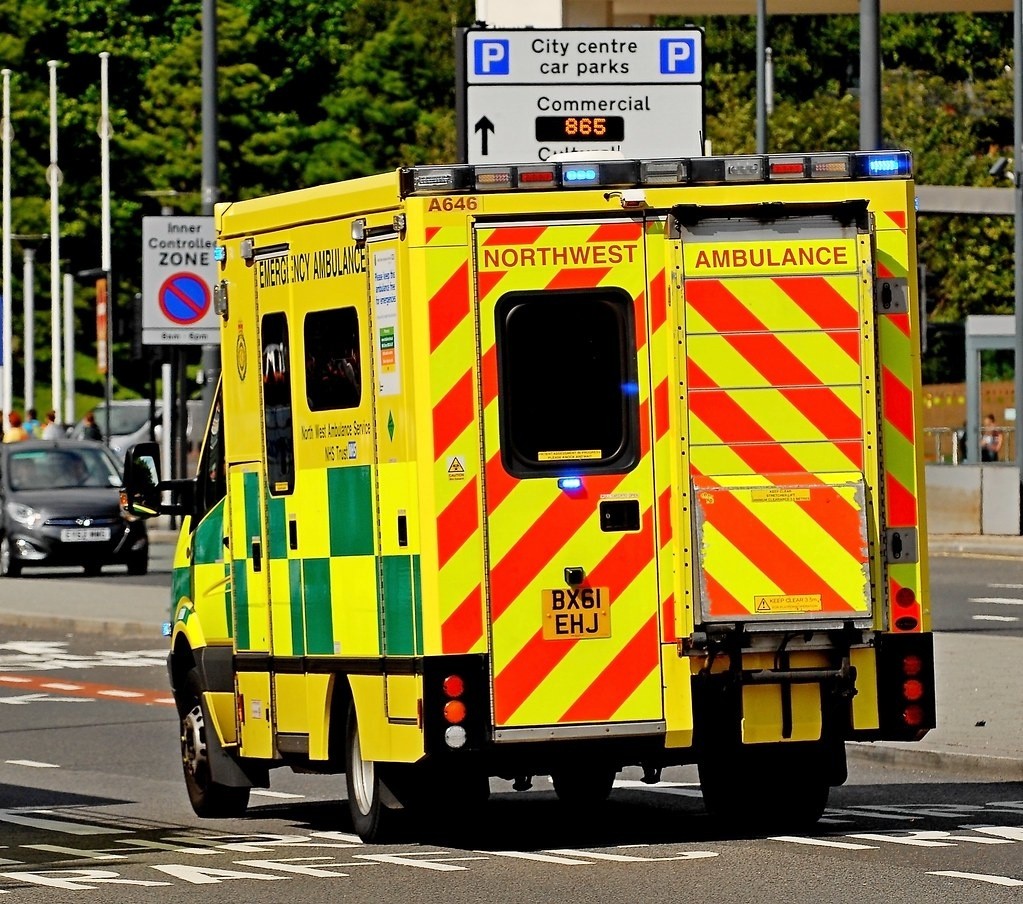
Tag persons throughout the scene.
[978,416,1002,461]
[21,411,41,444]
[76,412,102,442]
[956,419,975,459]
[3,412,31,445]
[42,412,67,442]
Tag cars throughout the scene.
[0,440,149,580]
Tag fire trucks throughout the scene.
[122,149,936,848]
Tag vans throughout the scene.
[64,401,207,475]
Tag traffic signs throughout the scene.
[465,27,706,167]
